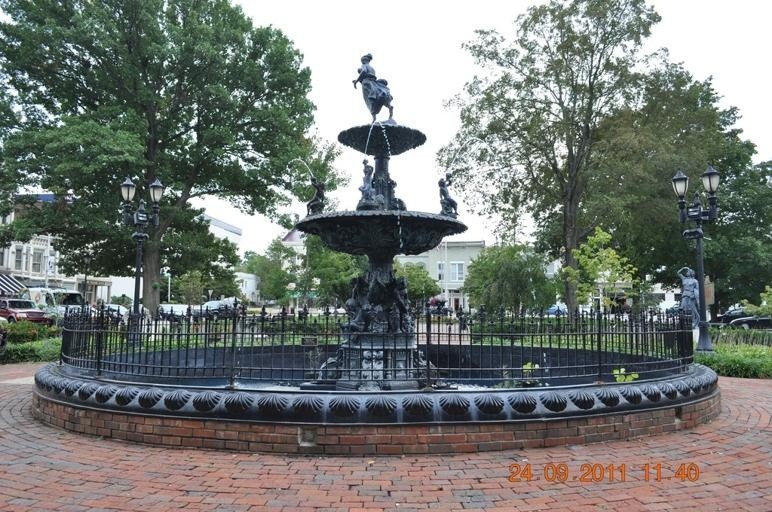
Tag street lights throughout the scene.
[122,176,162,344]
[671,167,722,350]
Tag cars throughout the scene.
[730,313,772,331]
[424,306,451,316]
[711,309,752,327]
[0,289,244,325]
[540,306,567,316]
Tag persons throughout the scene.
[352,53,393,124]
[358,160,373,199]
[305,177,324,218]
[438,177,459,215]
[676,267,700,330]
